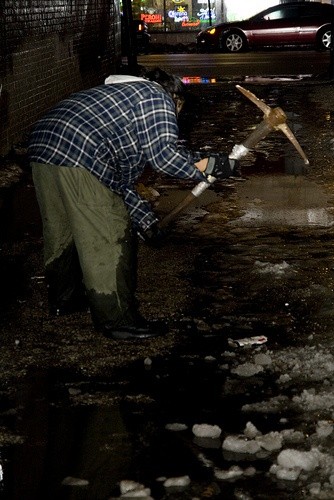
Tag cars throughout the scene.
[195,1,334,52]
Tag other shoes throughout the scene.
[47,283,90,316]
[102,308,159,339]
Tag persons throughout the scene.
[21,67,241,337]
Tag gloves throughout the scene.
[204,152,239,181]
